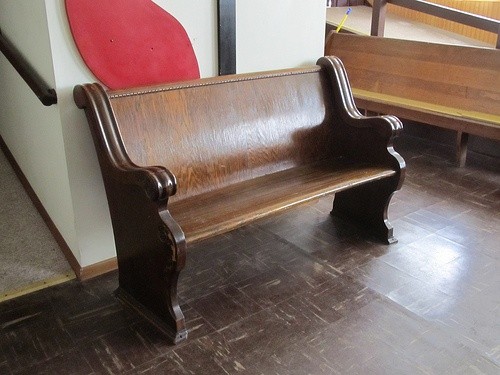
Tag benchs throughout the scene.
[324,28,500,167]
[70,55,409,347]
